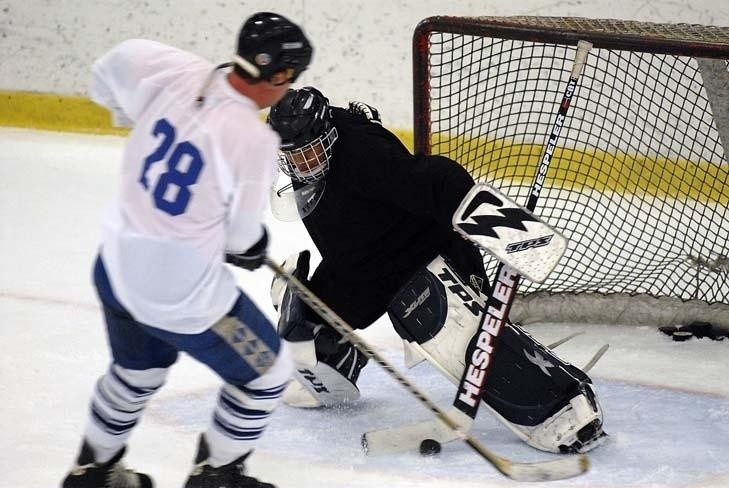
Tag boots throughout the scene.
[62,436,153,487]
[182,432,275,487]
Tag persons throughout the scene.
[62,11,313,487]
[263,86,511,383]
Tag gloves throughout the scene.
[224,224,269,272]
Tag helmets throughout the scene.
[233,11,312,83]
[267,85,334,152]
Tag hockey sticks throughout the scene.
[363,39,593,455]
[264,257,589,483]
